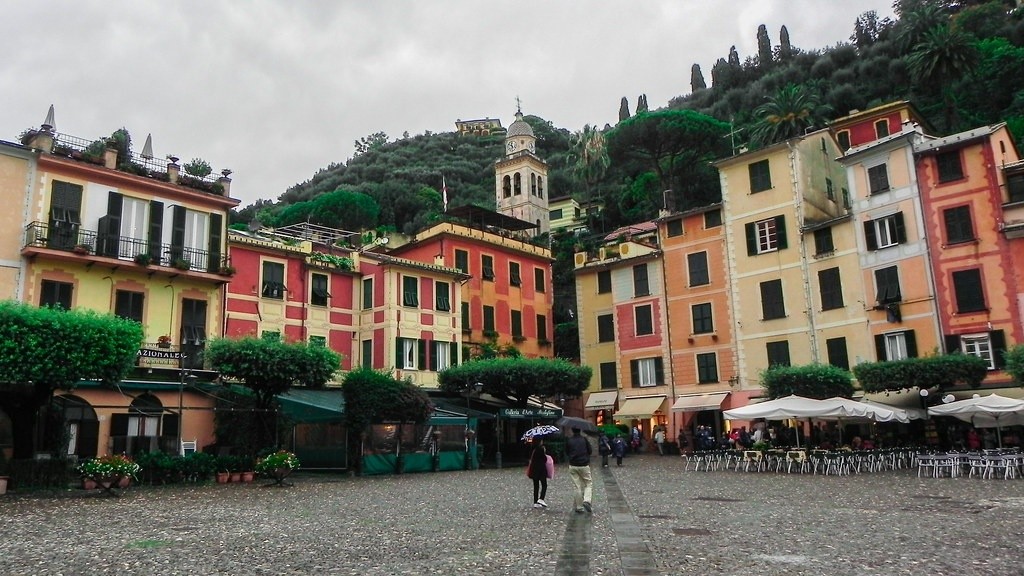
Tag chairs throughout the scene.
[680,444,1024,480]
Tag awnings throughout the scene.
[584,391,618,411]
[671,391,730,413]
[193,383,480,470]
[613,394,667,420]
[445,392,563,469]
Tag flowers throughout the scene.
[257,450,301,474]
[76,451,143,482]
[158,335,172,342]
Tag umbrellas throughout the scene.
[140,133,153,167]
[44,104,56,131]
[927,394,1024,449]
[556,416,599,432]
[816,396,930,443]
[723,393,866,449]
[521,424,560,440]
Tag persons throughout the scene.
[599,433,612,467]
[678,429,688,455]
[631,426,642,455]
[980,428,994,449]
[692,424,714,448]
[612,433,628,466]
[566,428,593,513]
[528,436,548,508]
[653,424,666,456]
[718,422,876,449]
[968,428,980,450]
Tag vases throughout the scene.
[113,477,130,487]
[268,467,291,477]
[159,342,171,348]
[97,481,112,488]
[82,477,96,489]
[0,475,9,495]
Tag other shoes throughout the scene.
[583,502,593,512]
[575,505,585,512]
[537,499,548,507]
[533,503,543,508]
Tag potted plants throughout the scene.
[215,265,236,277]
[169,258,191,271]
[134,253,153,266]
[72,243,92,255]
[216,454,255,483]
[19,130,228,196]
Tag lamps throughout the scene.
[181,336,188,345]
[688,332,693,341]
[712,329,719,338]
[727,376,738,387]
[195,337,201,346]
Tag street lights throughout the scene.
[176,338,201,455]
[920,388,932,450]
[467,374,484,439]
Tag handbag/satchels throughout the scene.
[526,459,532,476]
[729,437,736,444]
